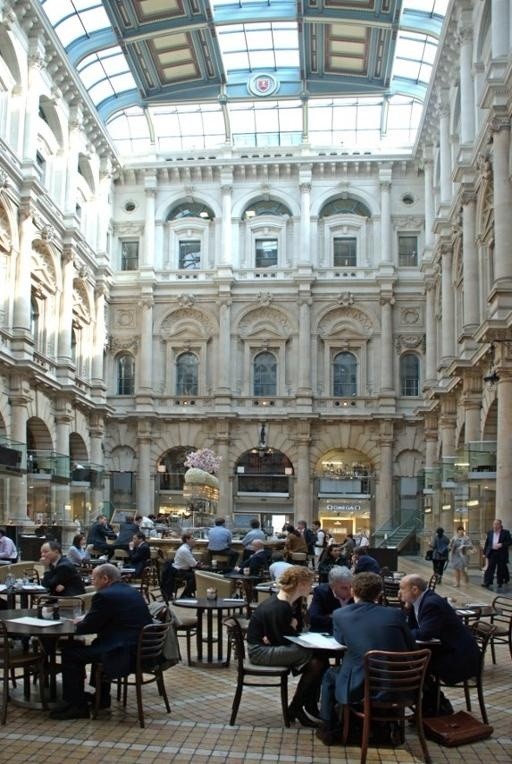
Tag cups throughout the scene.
[243,567,250,576]
[42,607,54,620]
[206,585,217,600]
[211,559,218,569]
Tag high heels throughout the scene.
[305,701,322,719]
[288,702,319,728]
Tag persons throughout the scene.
[427,528,450,584]
[502,555,509,584]
[481,519,510,587]
[448,527,473,587]
[316,572,419,746]
[398,574,482,723]
[0,515,372,720]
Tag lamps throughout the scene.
[284,462,293,476]
[236,462,245,474]
[158,462,167,473]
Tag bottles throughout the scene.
[5,566,17,589]
[53,601,60,620]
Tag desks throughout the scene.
[277,628,442,735]
[4,614,76,711]
[452,604,503,665]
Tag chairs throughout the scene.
[429,620,495,726]
[0,440,98,486]
[92,610,176,729]
[220,616,295,731]
[484,593,510,666]
[425,450,496,489]
[35,594,84,685]
[341,647,434,764]
[1,618,53,726]
[0,520,439,666]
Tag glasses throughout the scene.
[90,577,96,585]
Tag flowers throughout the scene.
[183,446,225,474]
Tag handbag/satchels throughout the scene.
[338,710,401,747]
[422,710,494,749]
[460,538,477,558]
[425,551,433,561]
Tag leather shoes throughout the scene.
[316,725,341,744]
[50,707,91,721]
[85,691,111,708]
[481,582,493,587]
